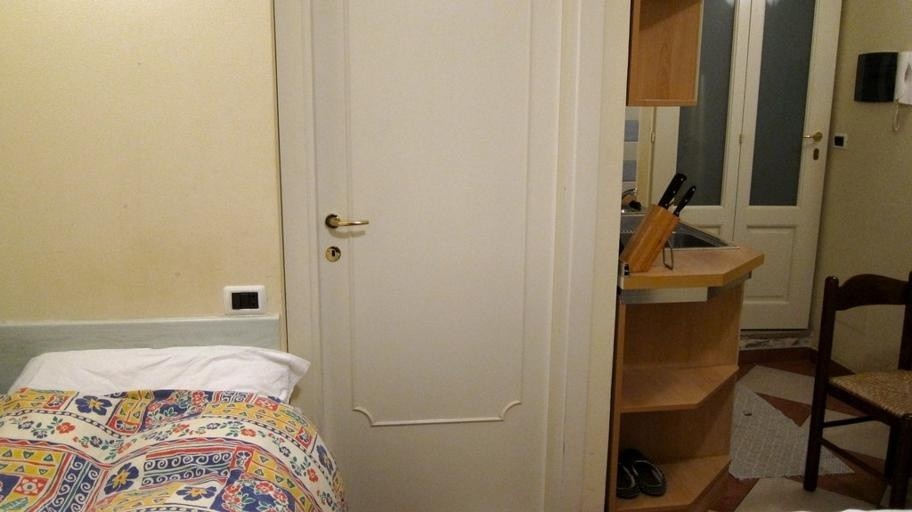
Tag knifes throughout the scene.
[657,172,698,219]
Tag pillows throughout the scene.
[8,347,311,405]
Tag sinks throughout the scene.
[622,225,730,254]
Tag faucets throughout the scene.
[622,184,639,205]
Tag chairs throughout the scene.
[802,269,912,509]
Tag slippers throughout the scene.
[617,449,666,499]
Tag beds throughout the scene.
[0,346,350,511]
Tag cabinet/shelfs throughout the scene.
[606,250,765,511]
[627,0,704,107]
